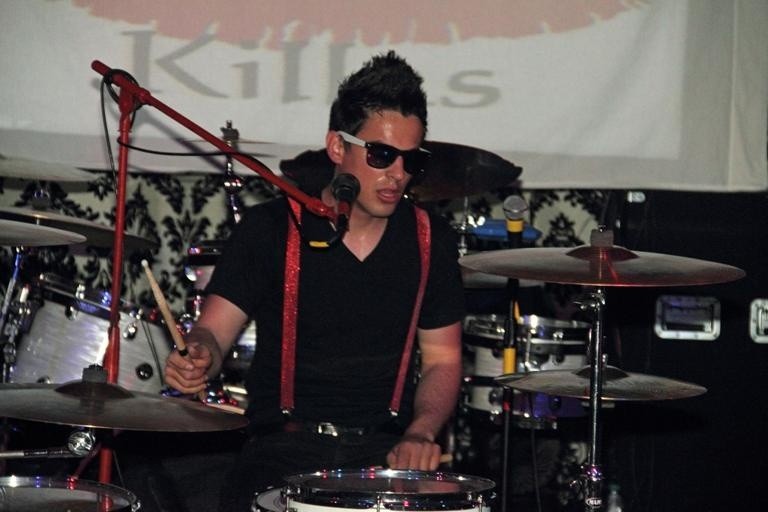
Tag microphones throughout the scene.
[67,428,96,458]
[499,318,518,413]
[501,195,527,302]
[328,172,360,246]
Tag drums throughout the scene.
[461,316,592,430]
[1,273,185,395]
[0,477,141,511]
[183,250,257,370]
[250,469,498,512]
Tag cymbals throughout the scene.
[0,206,158,250]
[0,380,250,433]
[495,364,708,401]
[279,140,523,202]
[457,246,748,287]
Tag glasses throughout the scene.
[336,131,433,181]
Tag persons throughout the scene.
[160,48,469,510]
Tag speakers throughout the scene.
[617,228,768,512]
